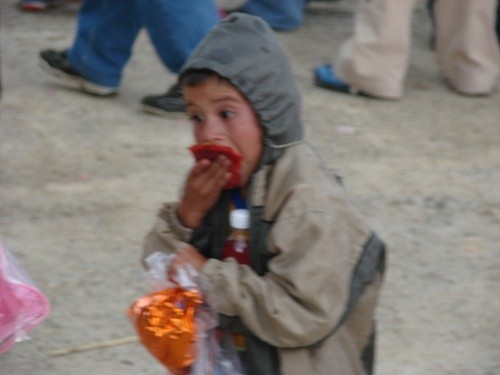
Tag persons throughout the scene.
[138,11,388,375]
[37,0,500,100]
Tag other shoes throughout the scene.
[19,0,47,12]
[314,64,383,99]
[219,8,239,21]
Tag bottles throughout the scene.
[214,209,253,352]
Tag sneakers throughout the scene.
[37,47,119,95]
[140,82,190,120]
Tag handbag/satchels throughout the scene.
[123,250,243,375]
